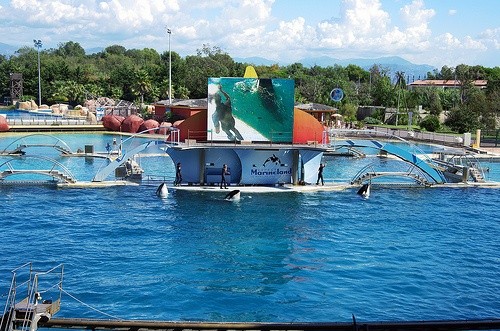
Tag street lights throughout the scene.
[167,28,171,106]
[33,39,43,106]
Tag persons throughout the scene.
[220,164,230,189]
[317,163,325,185]
[175,163,182,186]
[212,85,243,140]
